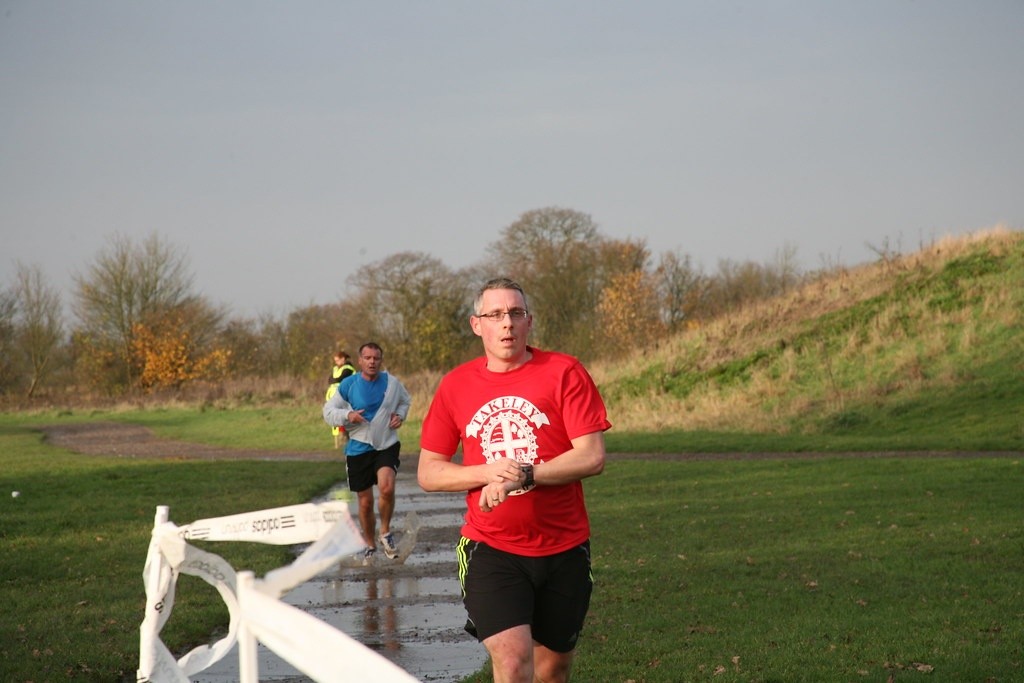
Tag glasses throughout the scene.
[476,308,529,322]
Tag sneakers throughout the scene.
[362,547,376,567]
[378,532,399,559]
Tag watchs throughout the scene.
[518,463,536,491]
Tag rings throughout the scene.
[492,498,498,501]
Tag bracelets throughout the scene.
[397,414,403,422]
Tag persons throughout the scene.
[326,351,356,441]
[322,343,411,566]
[417,273,613,683]
